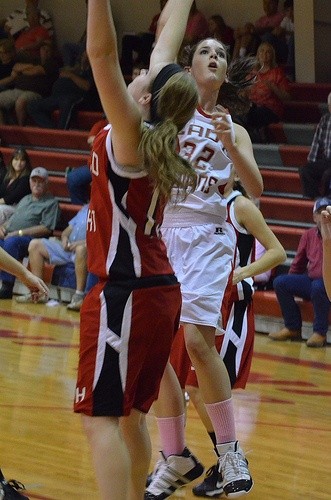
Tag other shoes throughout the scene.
[0,280,14,299]
[268,326,302,342]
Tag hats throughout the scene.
[313,197,331,214]
[30,167,48,184]
[66,166,91,204]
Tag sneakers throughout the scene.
[144,447,203,500]
[0,469,29,500]
[66,294,85,311]
[283,340,288,341]
[193,448,248,498]
[307,330,327,347]
[16,290,48,303]
[145,458,165,488]
[214,440,254,497]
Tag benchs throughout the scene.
[0,75,331,343]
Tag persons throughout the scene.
[0,146,35,225]
[146,0,263,500]
[14,199,89,312]
[275,198,330,347]
[300,93,331,200]
[0,167,63,298]
[0,0,292,130]
[192,173,286,500]
[73,1,182,500]
